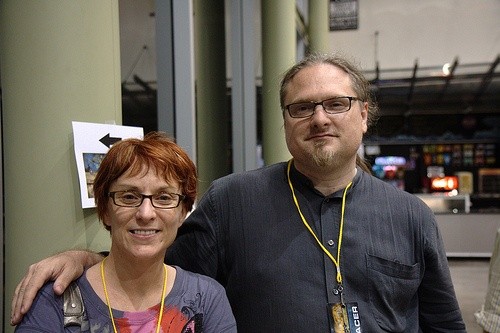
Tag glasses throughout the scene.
[281,96,362,118]
[108,190,189,209]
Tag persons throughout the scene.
[13,131,237,333]
[11,52,467,333]
